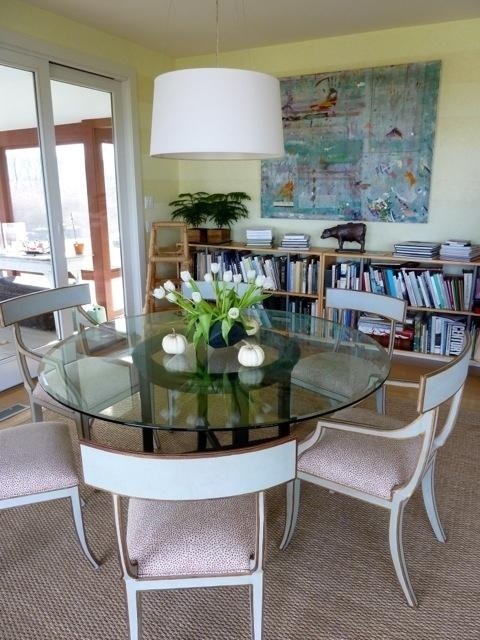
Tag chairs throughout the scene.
[2,423,102,571]
[79,441,296,639]
[180,279,263,309]
[277,329,475,611]
[288,286,409,420]
[0,282,138,442]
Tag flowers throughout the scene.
[154,261,272,343]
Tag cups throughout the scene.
[73,242,85,255]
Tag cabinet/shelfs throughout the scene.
[196,245,327,318]
[321,248,478,365]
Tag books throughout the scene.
[246,226,274,248]
[393,240,480,262]
[281,233,311,250]
[196,248,320,295]
[288,298,319,335]
[246,304,273,328]
[323,260,480,363]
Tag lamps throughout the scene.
[146,1,289,162]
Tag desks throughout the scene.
[38,308,391,459]
[1,239,92,340]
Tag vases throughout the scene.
[202,317,245,349]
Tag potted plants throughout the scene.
[172,190,211,245]
[203,191,251,244]
[68,212,86,255]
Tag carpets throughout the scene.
[1,341,479,640]
